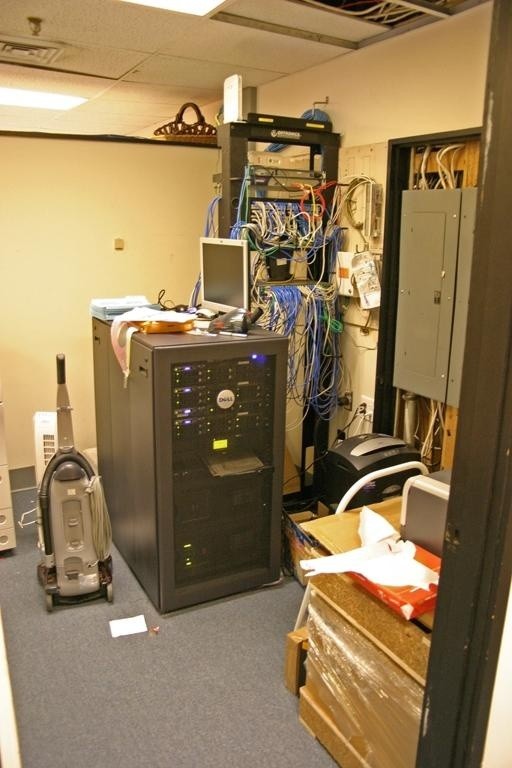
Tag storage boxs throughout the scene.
[280,500,333,588]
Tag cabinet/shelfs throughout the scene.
[392,187,478,409]
[89,315,283,614]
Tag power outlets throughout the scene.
[358,394,374,424]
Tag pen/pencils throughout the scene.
[219,331,247,339]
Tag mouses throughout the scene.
[196,307,213,319]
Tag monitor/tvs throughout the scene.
[199,235,250,317]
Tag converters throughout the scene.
[339,398,349,405]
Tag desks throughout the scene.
[287,547,430,768]
